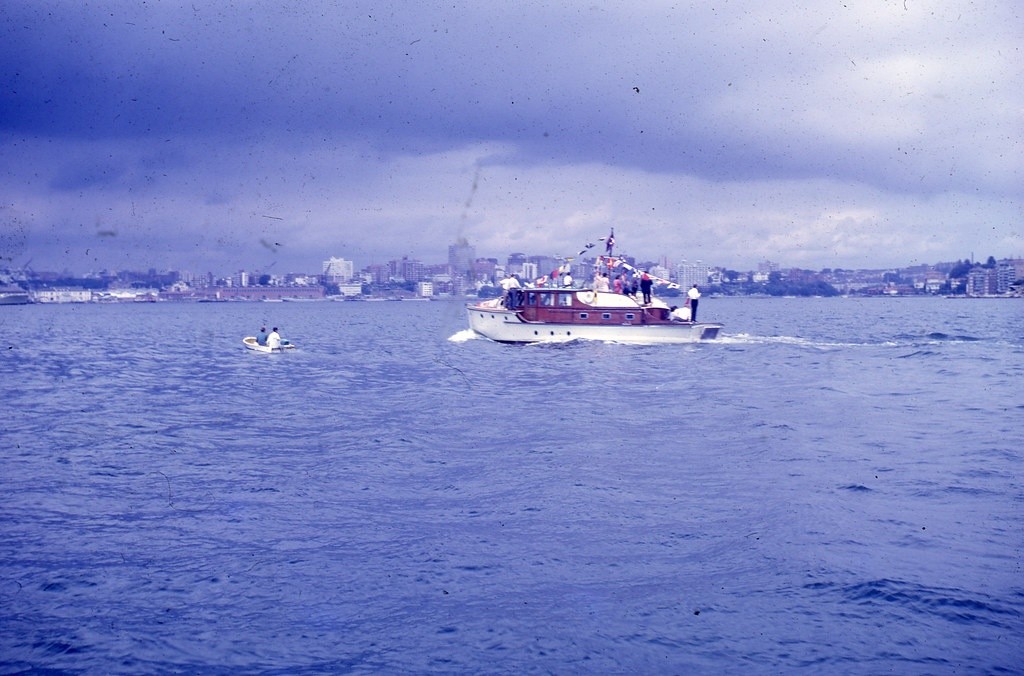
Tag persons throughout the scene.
[687,284,701,322]
[640,274,653,303]
[564,272,573,286]
[256,327,268,346]
[595,272,609,292]
[267,327,281,346]
[613,272,638,295]
[501,273,521,304]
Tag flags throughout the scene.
[536,257,575,288]
[601,255,634,272]
[578,236,615,255]
[633,269,681,290]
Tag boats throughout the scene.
[466,226,726,346]
[0,280,30,306]
[243,336,295,354]
